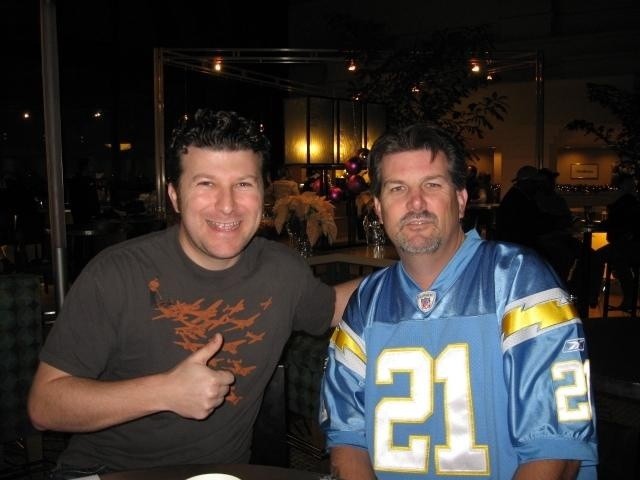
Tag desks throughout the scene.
[569,223,608,247]
[41,215,165,292]
[466,203,500,242]
[307,245,401,285]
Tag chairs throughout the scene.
[252,331,329,465]
[2,272,46,470]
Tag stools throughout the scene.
[603,258,638,321]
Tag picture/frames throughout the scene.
[569,163,599,179]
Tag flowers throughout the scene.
[271,191,339,247]
[354,190,377,220]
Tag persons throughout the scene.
[460,166,640,318]
[303,171,321,191]
[27,109,366,480]
[317,120,599,480]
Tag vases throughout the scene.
[288,229,315,258]
[363,216,386,248]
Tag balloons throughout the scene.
[311,156,366,203]
